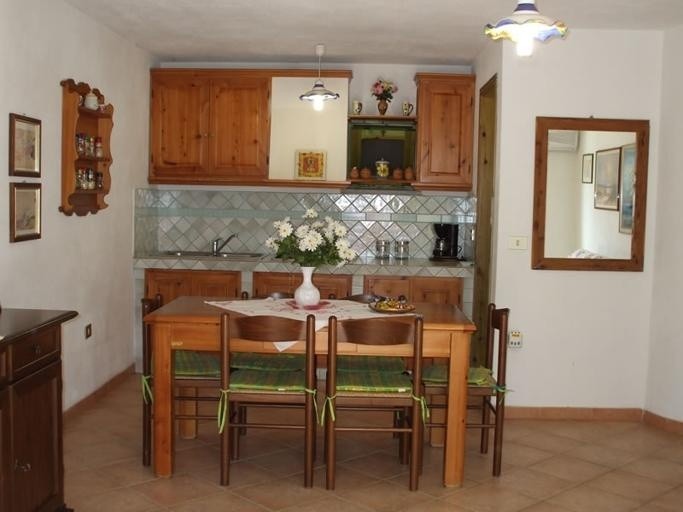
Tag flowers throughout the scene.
[264,208,356,265]
[370,77,398,102]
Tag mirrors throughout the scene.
[530,116,652,272]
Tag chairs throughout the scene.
[138,295,249,462]
[400,300,510,479]
[316,315,419,492]
[214,310,320,488]
[238,289,337,433]
[325,290,410,463]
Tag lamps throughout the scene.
[483,0,567,56]
[297,43,340,112]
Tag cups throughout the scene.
[401,100,414,116]
[351,101,363,115]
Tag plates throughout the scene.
[368,301,416,313]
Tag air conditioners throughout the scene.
[547,129,578,153]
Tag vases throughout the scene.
[378,99,389,115]
[294,266,320,307]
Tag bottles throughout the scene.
[374,239,391,259]
[83,90,99,111]
[350,166,371,179]
[75,133,105,157]
[392,165,415,181]
[375,157,390,178]
[75,167,105,191]
[394,240,409,259]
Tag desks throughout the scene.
[143,295,478,490]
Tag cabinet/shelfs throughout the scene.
[143,268,242,304]
[363,275,464,312]
[413,73,475,192]
[252,271,353,300]
[349,113,416,184]
[149,67,271,185]
[1,309,80,510]
[61,79,116,218]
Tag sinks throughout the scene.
[192,251,270,262]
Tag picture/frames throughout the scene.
[9,180,42,244]
[7,112,42,178]
[618,141,635,234]
[593,147,622,210]
[581,153,593,184]
[295,150,326,180]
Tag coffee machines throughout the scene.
[428,221,459,260]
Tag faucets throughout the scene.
[211,233,238,256]
